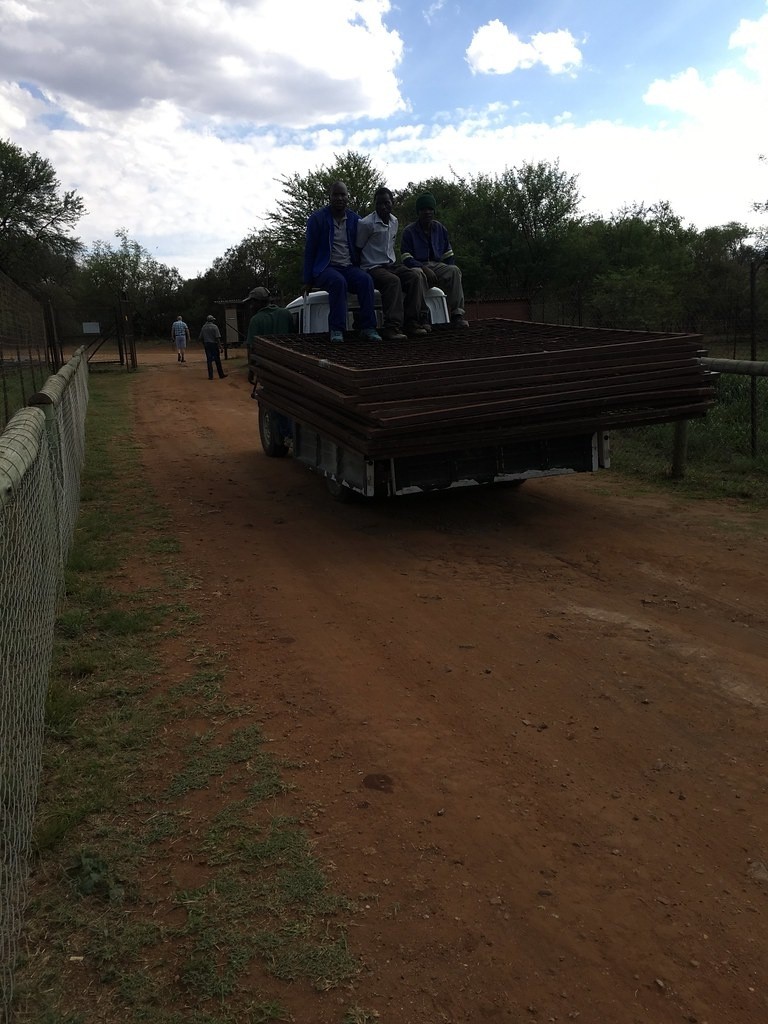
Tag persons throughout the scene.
[196,315,229,380]
[302,180,470,345]
[171,316,191,363]
[246,286,294,384]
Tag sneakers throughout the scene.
[422,325,432,333]
[452,318,469,327]
[360,328,382,341]
[407,325,427,334]
[383,327,408,340]
[330,330,344,342]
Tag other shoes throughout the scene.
[208,378,213,380]
[181,360,186,362]
[220,374,228,378]
[178,356,181,361]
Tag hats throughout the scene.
[207,315,216,321]
[242,287,270,302]
[416,191,436,215]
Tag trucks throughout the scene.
[245,285,613,502]
